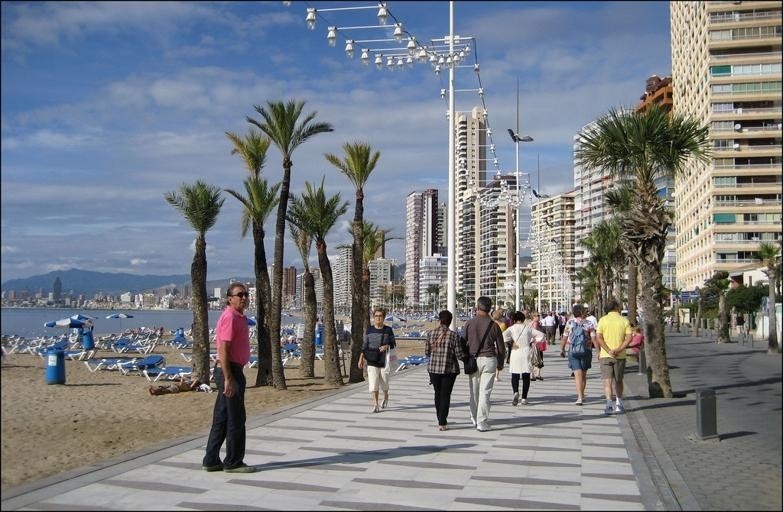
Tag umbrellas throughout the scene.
[246,315,255,326]
[62,313,99,343]
[104,313,134,330]
[44,316,95,340]
[385,314,407,327]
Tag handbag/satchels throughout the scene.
[540,340,548,352]
[363,347,380,362]
[464,355,477,374]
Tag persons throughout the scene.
[491,304,645,415]
[148,373,201,396]
[202,282,258,473]
[424,310,469,431]
[464,296,505,432]
[357,307,397,413]
[212,359,225,390]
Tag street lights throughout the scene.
[532,189,572,313]
[507,129,534,311]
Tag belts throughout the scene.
[213,360,229,368]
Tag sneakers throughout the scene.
[372,399,388,414]
[223,464,256,473]
[576,395,586,408]
[471,418,491,431]
[439,419,448,432]
[202,462,223,471]
[512,392,530,406]
[532,376,541,381]
[606,403,625,415]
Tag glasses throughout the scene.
[229,292,249,298]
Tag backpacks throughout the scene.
[570,320,587,356]
